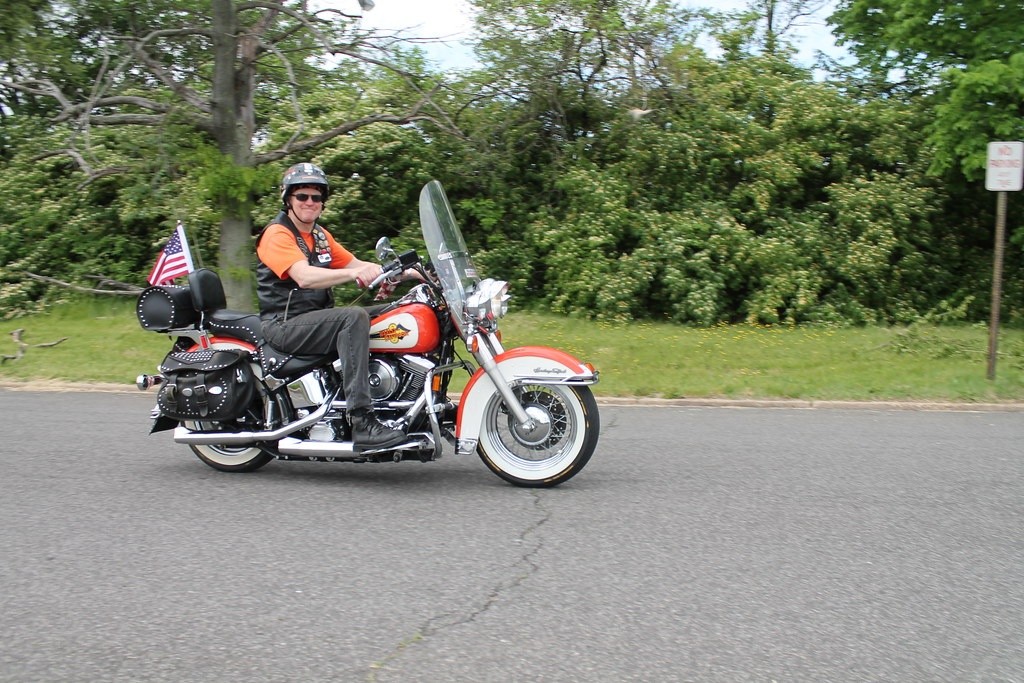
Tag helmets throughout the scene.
[281,162,330,204]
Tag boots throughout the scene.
[351,411,407,452]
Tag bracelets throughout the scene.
[408,268,412,279]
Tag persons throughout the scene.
[254,162,430,451]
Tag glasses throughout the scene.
[288,193,323,202]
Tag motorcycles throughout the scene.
[128,179,601,489]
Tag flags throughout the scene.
[147,228,188,286]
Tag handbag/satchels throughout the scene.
[156,349,254,421]
